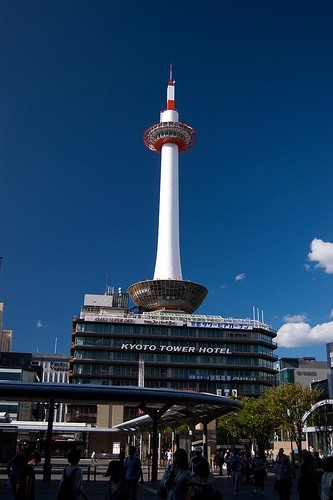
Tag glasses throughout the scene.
[174,453,181,457]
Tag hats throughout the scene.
[104,461,116,477]
[195,447,202,455]
[226,450,230,453]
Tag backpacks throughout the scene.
[57,468,80,500]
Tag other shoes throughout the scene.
[252,492,257,496]
[233,491,240,496]
[262,493,264,496]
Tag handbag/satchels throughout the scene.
[157,465,175,499]
[273,464,293,495]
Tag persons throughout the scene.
[103,446,144,500]
[56,448,89,500]
[298,455,322,500]
[273,472,293,500]
[158,449,192,500]
[318,456,333,500]
[214,447,273,496]
[5,412,10,423]
[149,438,208,474]
[7,449,35,500]
[276,449,333,483]
[185,456,219,500]
[91,449,97,467]
